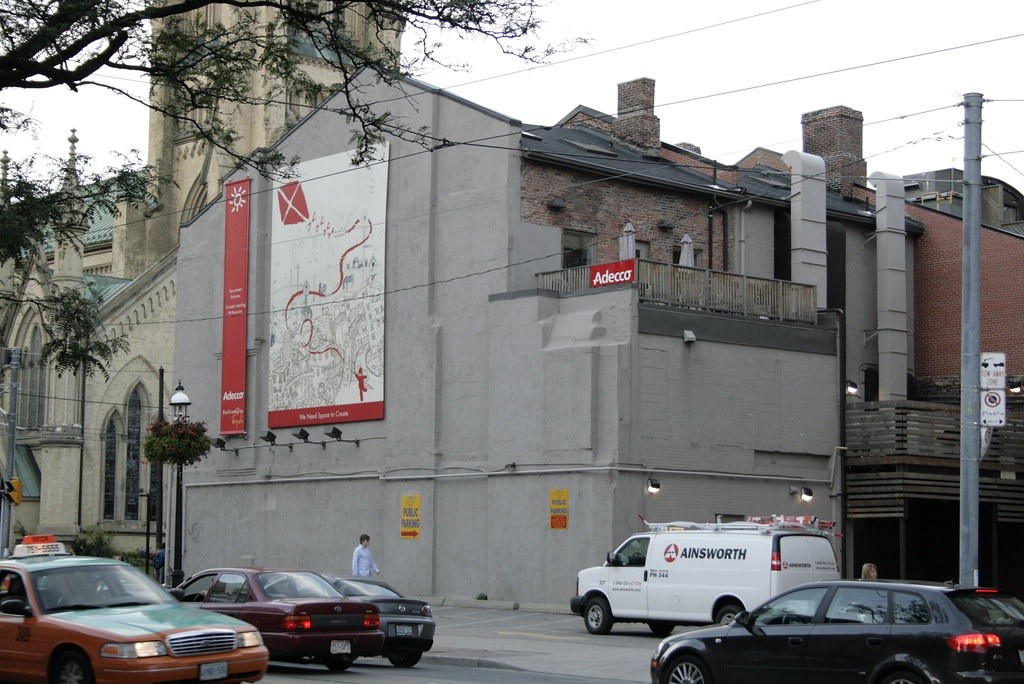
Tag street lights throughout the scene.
[169,379,191,588]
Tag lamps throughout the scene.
[684,329,696,344]
[549,199,567,208]
[258,430,277,445]
[1009,380,1021,393]
[208,438,226,450]
[847,380,857,394]
[323,427,343,441]
[291,428,309,443]
[658,220,675,229]
[802,481,813,502]
[647,473,660,493]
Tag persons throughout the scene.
[352,534,381,577]
[861,563,877,580]
[157,543,165,583]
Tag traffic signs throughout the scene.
[980,391,1006,427]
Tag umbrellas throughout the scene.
[618,223,635,260]
[678,233,694,273]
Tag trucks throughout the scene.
[571,513,844,636]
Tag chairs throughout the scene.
[7,576,45,612]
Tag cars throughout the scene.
[176,568,385,671]
[651,579,1024,684]
[0,534,270,684]
[321,574,435,668]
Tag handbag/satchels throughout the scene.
[154,553,163,569]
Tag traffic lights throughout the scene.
[4,480,22,505]
